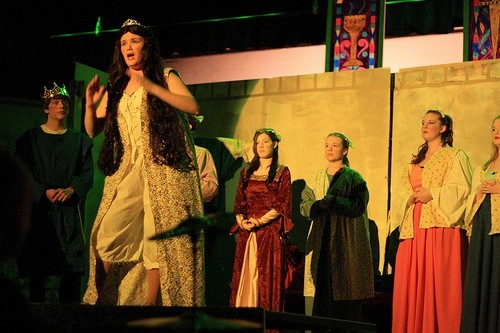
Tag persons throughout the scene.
[460,115,500,333]
[13,83,94,306]
[186,113,219,203]
[228,128,296,333]
[299,132,375,321]
[392,109,473,333]
[80,18,206,307]
